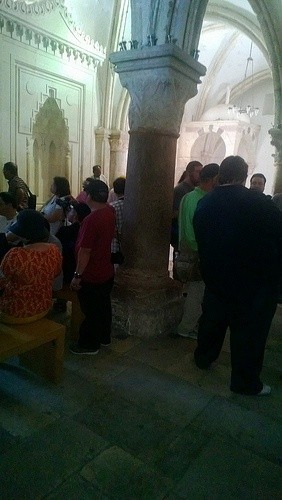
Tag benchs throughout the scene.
[0,319,65,384]
[52,287,86,325]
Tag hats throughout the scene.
[82,180,108,201]
[7,209,50,243]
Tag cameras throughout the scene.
[56,197,71,214]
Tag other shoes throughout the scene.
[178,328,198,339]
[70,344,99,355]
[230,383,272,396]
[99,337,112,346]
[193,353,225,370]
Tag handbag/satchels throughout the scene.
[55,226,67,241]
[174,248,205,282]
[28,195,36,210]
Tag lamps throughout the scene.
[227,42,260,120]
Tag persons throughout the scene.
[0,156,282,395]
